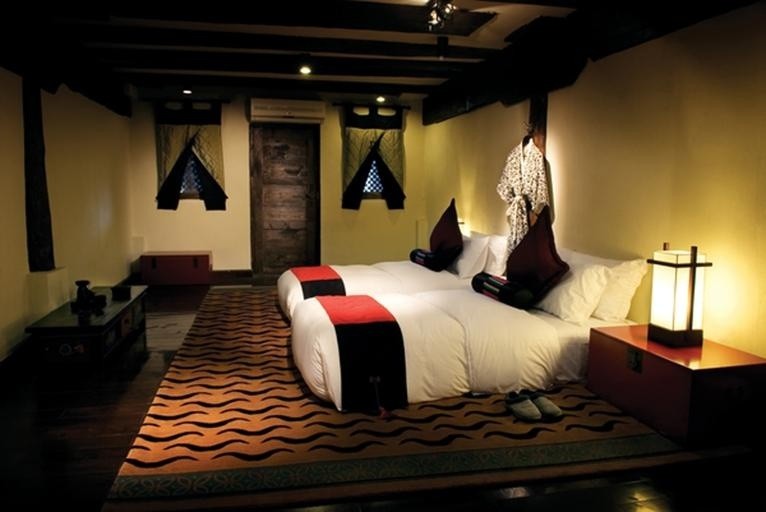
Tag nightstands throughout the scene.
[585,322,766,441]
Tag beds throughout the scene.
[276,227,511,326]
[289,242,651,417]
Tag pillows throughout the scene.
[535,261,614,326]
[470,271,514,302]
[469,229,514,278]
[409,247,442,273]
[555,245,650,323]
[455,228,493,282]
[428,196,465,274]
[508,203,570,313]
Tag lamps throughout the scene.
[647,242,715,350]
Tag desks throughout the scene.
[140,251,212,286]
[22,284,147,386]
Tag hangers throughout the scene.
[519,120,533,146]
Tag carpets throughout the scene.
[98,286,765,512]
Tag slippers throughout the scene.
[505,389,562,420]
